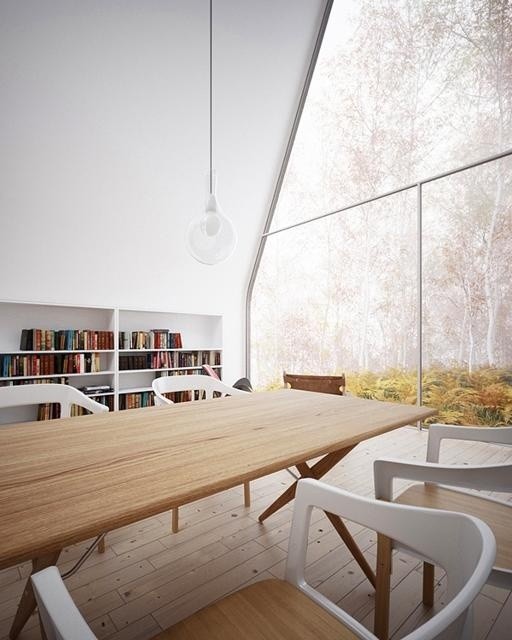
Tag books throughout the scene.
[1,329,221,420]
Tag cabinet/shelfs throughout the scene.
[0,301,224,419]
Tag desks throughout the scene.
[0,388,440,640]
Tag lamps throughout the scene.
[185,0,237,265]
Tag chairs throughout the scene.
[29,477,497,640]
[0,384,110,553]
[282,371,346,396]
[373,422,512,640]
[152,374,253,533]
